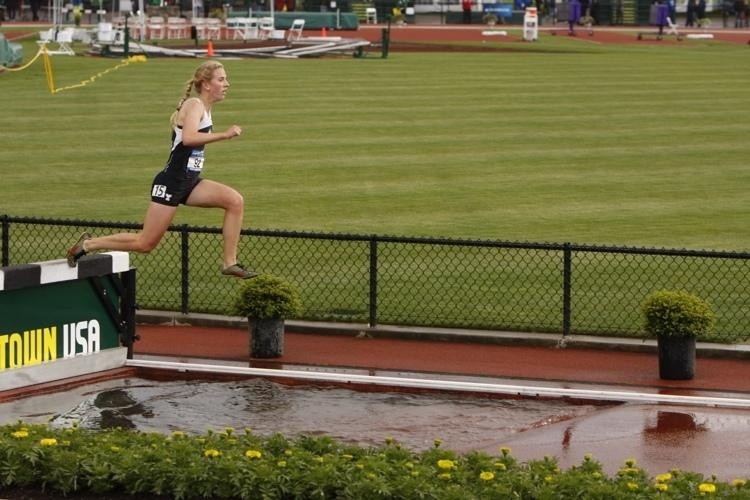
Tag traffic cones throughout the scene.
[322,28,326,36]
[206,39,214,56]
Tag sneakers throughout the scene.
[221,263,258,280]
[67,231,91,268]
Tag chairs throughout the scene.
[33,15,306,58]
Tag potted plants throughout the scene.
[231,270,301,360]
[639,287,713,380]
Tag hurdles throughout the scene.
[1,250,138,393]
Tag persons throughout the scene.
[63,60,259,279]
